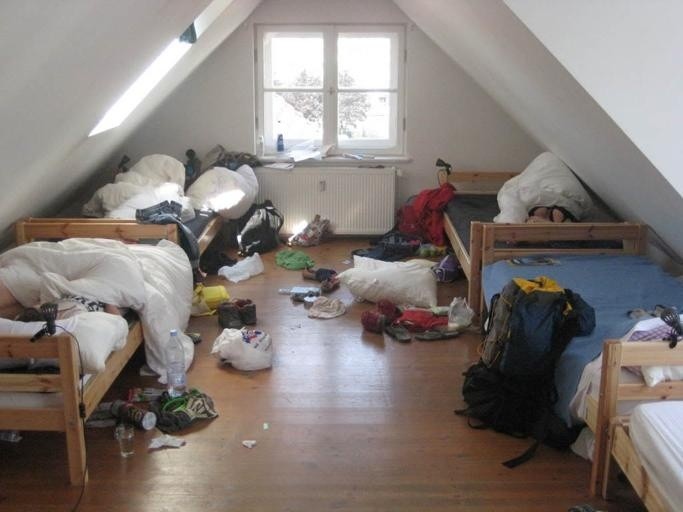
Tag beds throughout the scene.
[597,341,681,512]
[23,170,230,266]
[0,219,177,485]
[435,168,636,316]
[480,222,680,498]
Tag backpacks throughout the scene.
[235,200,283,256]
[454,276,595,467]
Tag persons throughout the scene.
[528,206,569,223]
[0,279,125,329]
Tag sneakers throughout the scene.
[218,298,256,328]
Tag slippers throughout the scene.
[361,299,458,342]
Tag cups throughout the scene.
[116,423,135,459]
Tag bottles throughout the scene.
[275,120,284,154]
[164,329,187,399]
[256,135,264,157]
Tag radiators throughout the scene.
[251,166,396,237]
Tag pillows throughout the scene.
[0,311,129,374]
[333,255,436,308]
